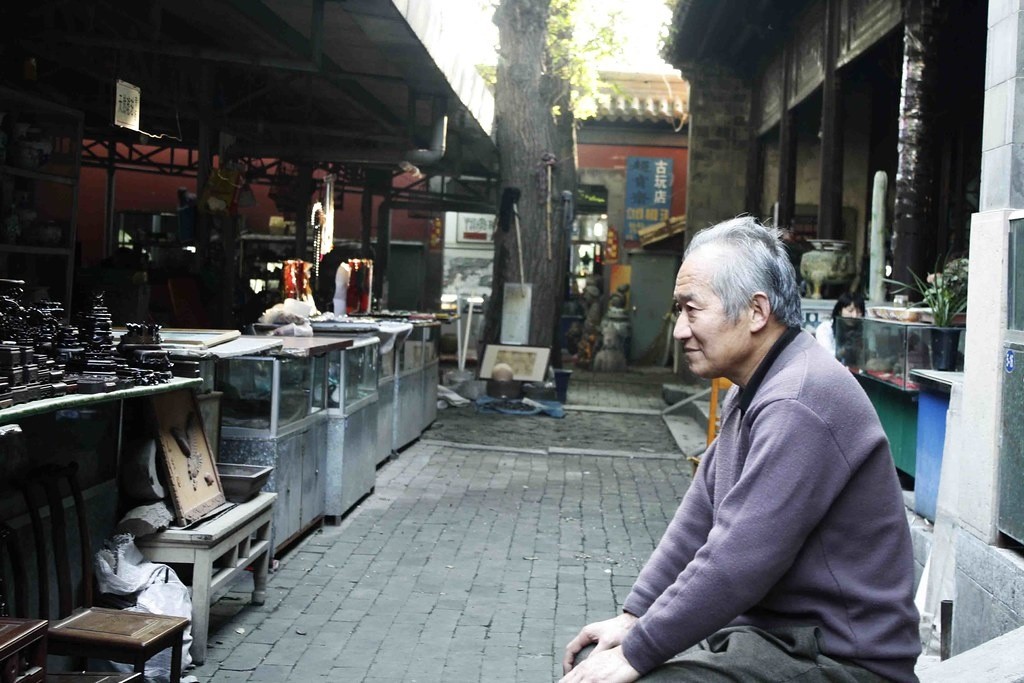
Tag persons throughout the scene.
[556,210,920,683]
[814,291,866,359]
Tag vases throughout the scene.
[215,463,273,504]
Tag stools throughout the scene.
[0,617,49,683]
[134,491,279,667]
[45,672,143,683]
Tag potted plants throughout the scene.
[881,243,968,371]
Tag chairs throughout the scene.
[0,463,190,683]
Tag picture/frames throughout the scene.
[145,388,226,527]
[475,343,552,383]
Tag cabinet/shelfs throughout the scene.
[0,77,85,326]
[205,351,329,554]
[376,320,440,466]
[0,397,123,671]
[324,336,382,526]
[835,315,966,479]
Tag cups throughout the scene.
[894,295,909,307]
